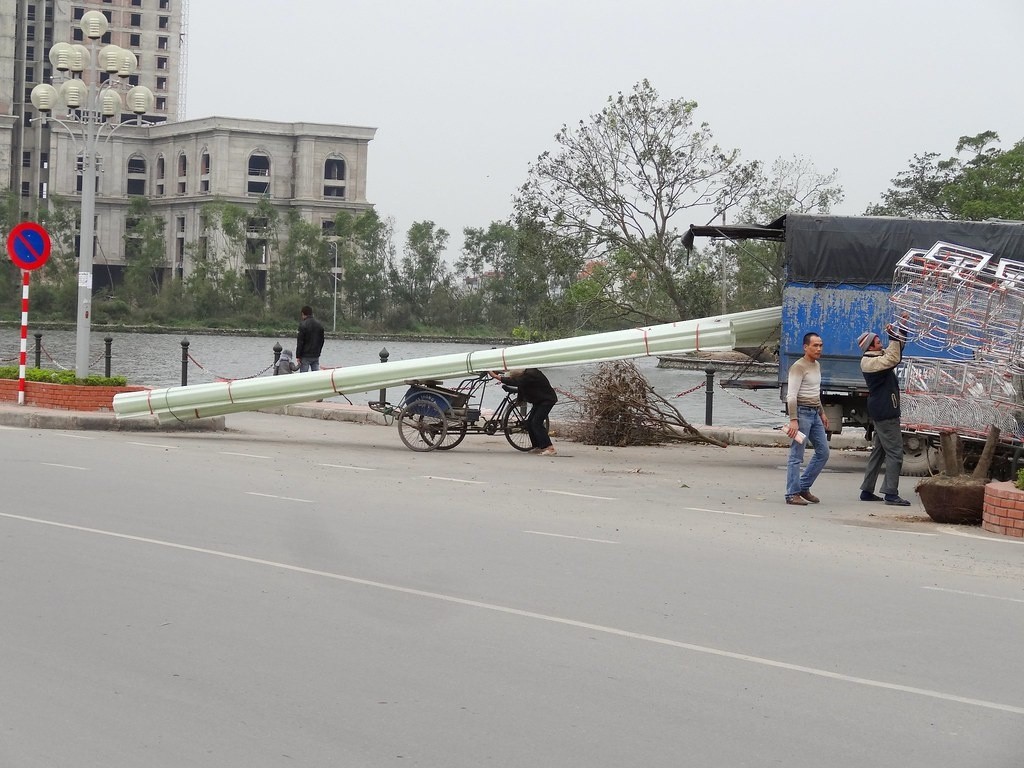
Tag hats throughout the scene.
[283,350,292,357]
[856,333,876,353]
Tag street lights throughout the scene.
[331,242,338,331]
[31,11,156,379]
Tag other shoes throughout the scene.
[528,448,548,453]
[885,495,911,505]
[788,495,808,505]
[801,491,820,503]
[538,450,557,457]
[860,492,884,501]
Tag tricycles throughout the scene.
[395,370,550,452]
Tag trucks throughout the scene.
[719,212,1024,477]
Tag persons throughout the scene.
[295,306,325,403]
[856,311,911,507]
[273,349,301,376]
[784,332,830,505]
[488,368,559,455]
